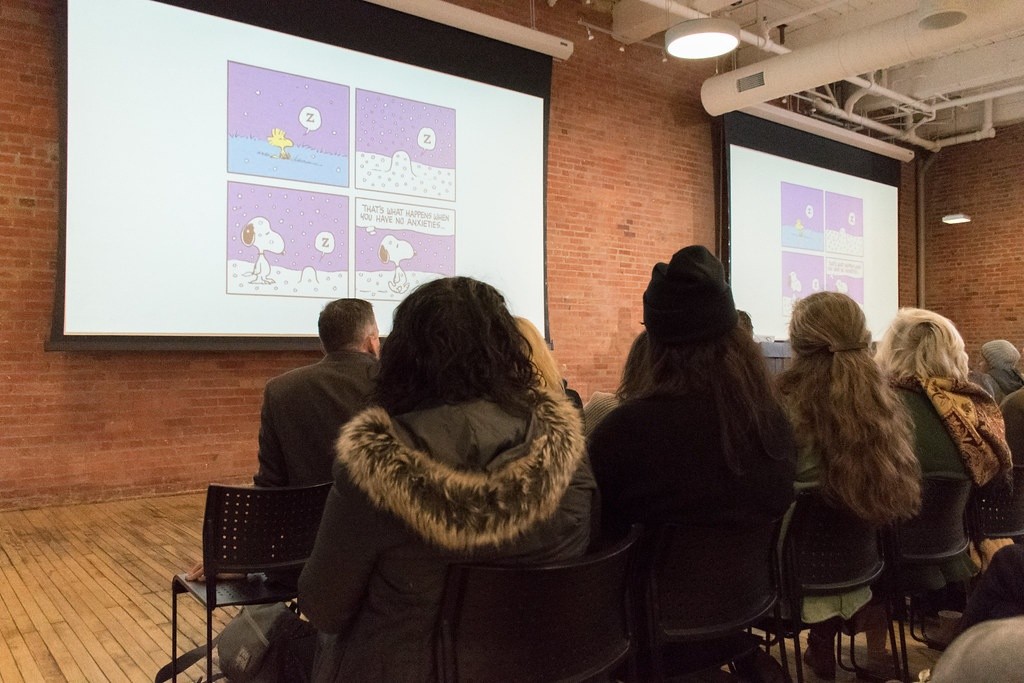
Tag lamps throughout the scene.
[665,17,741,60]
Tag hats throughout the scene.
[981,340,1021,370]
[642,245,739,347]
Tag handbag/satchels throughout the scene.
[218,600,303,683]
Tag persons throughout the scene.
[183,297,383,590]
[582,244,798,683]
[865,306,976,677]
[929,339,1024,683]
[295,276,602,683]
[766,292,923,683]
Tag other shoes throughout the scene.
[801,633,836,669]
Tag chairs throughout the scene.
[893,476,976,682]
[433,520,648,682]
[646,496,790,683]
[970,465,1024,538]
[755,486,903,683]
[171,479,335,683]
